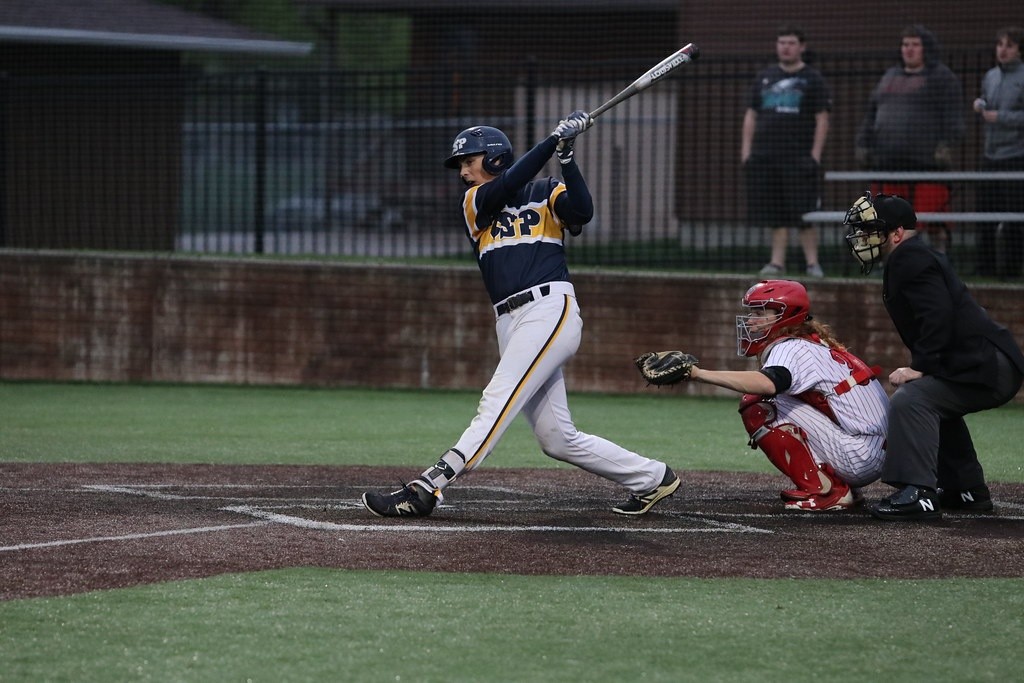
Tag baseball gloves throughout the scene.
[633,349,694,388]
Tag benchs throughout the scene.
[803,211,1024,222]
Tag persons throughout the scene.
[635,280,888,509]
[844,191,1024,518]
[741,29,834,276]
[973,27,1024,276]
[363,110,681,517]
[860,26,961,255]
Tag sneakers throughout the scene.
[871,483,945,521]
[783,483,853,512]
[779,488,810,502]
[936,486,992,512]
[612,464,681,515]
[361,478,436,518]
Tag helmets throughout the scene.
[843,190,918,276]
[735,279,810,359]
[443,125,514,175]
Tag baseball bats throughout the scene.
[557,41,701,140]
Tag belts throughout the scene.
[497,285,549,316]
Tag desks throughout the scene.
[825,171,1024,205]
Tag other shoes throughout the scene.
[803,265,825,278]
[759,263,785,279]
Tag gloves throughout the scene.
[566,109,593,133]
[554,119,574,158]
